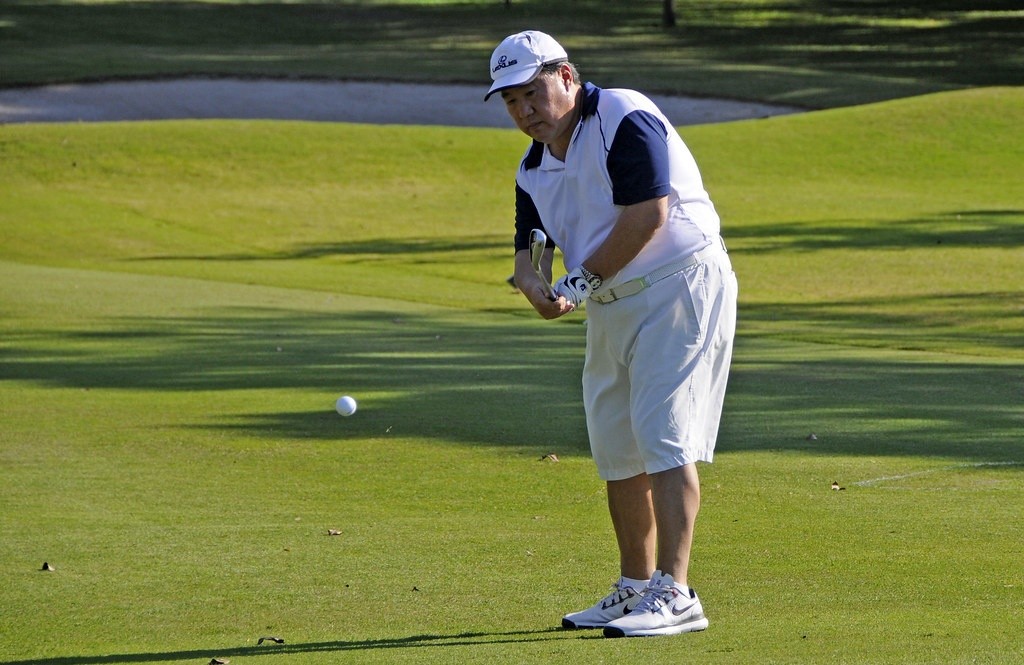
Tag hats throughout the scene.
[484,30,568,101]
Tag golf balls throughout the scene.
[336,395,357,417]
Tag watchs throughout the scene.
[577,264,604,291]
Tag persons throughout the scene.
[485,29,738,637]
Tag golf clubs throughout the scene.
[528,228,559,301]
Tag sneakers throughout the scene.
[562,571,709,636]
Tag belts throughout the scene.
[589,239,724,303]
